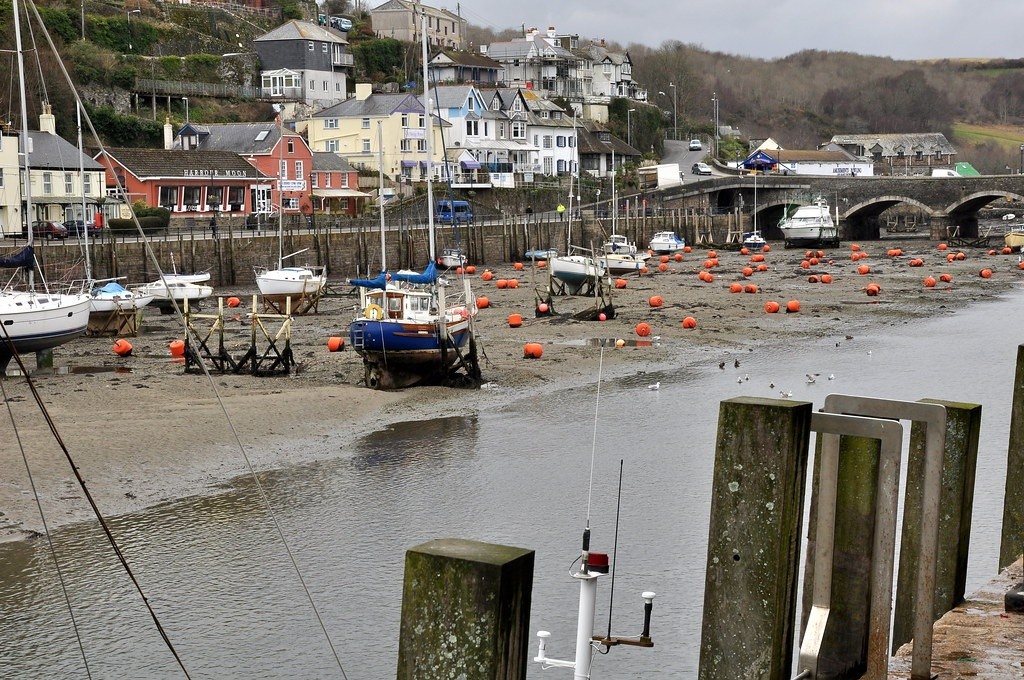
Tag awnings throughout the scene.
[401,160,417,168]
[420,161,434,168]
[461,160,481,169]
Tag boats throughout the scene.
[524,248,560,260]
[124,252,214,305]
[649,231,686,253]
[777,190,840,247]
[1003,224,1024,249]
[439,246,468,269]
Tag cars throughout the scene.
[23,220,70,240]
[62,219,100,239]
[691,162,712,175]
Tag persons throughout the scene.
[557,203,565,222]
[335,218,340,228]
[526,206,533,221]
[209,217,217,238]
[739,167,742,174]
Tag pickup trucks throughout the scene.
[688,139,702,150]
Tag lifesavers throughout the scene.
[366,304,381,319]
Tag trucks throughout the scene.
[436,201,476,225]
[640,163,684,191]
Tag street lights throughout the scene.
[669,85,677,140]
[736,151,741,175]
[776,146,781,174]
[182,99,189,123]
[1019,144,1024,176]
[711,98,719,160]
[627,108,635,146]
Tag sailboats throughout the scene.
[349,7,494,387]
[743,168,770,250]
[533,108,606,295]
[57,99,157,311]
[255,105,329,294]
[597,151,652,274]
[0,0,92,352]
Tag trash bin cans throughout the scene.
[645,208,652,216]
[696,208,704,216]
[292,215,300,228]
[305,213,315,229]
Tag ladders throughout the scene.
[354,322,364,350]
